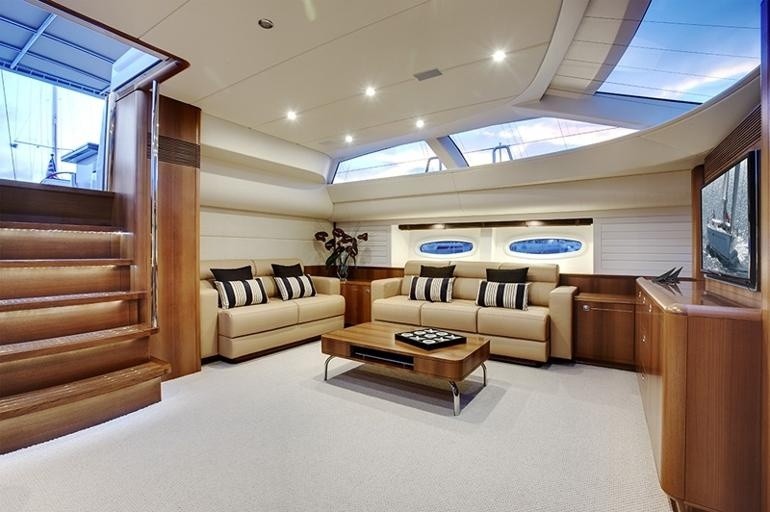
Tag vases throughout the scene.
[336,265,349,281]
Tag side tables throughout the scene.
[340,279,371,326]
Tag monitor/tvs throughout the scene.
[699,149,760,293]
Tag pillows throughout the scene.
[210,263,317,310]
[407,264,457,311]
[475,267,533,311]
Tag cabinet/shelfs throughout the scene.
[634,278,763,512]
[321,322,491,415]
[574,292,635,372]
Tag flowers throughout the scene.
[315,227,368,276]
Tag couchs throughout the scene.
[372,260,579,367]
[200,259,346,364]
